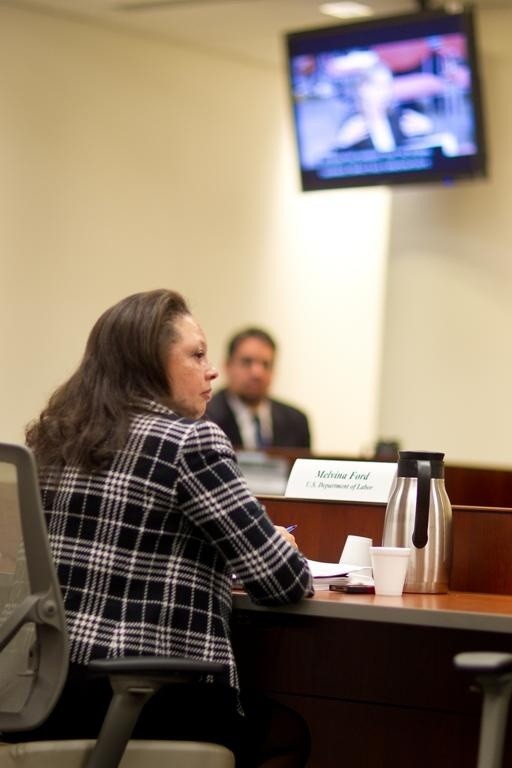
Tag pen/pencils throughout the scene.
[285,524,298,533]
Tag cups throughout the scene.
[338,535,373,568]
[369,547,411,597]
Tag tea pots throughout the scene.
[382,452,453,595]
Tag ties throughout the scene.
[252,416,262,444]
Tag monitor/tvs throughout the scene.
[287,13,486,191]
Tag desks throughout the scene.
[229,496,511,768]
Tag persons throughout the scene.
[27,287,313,768]
[204,328,312,450]
[328,40,471,147]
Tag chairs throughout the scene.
[1,441,240,766]
